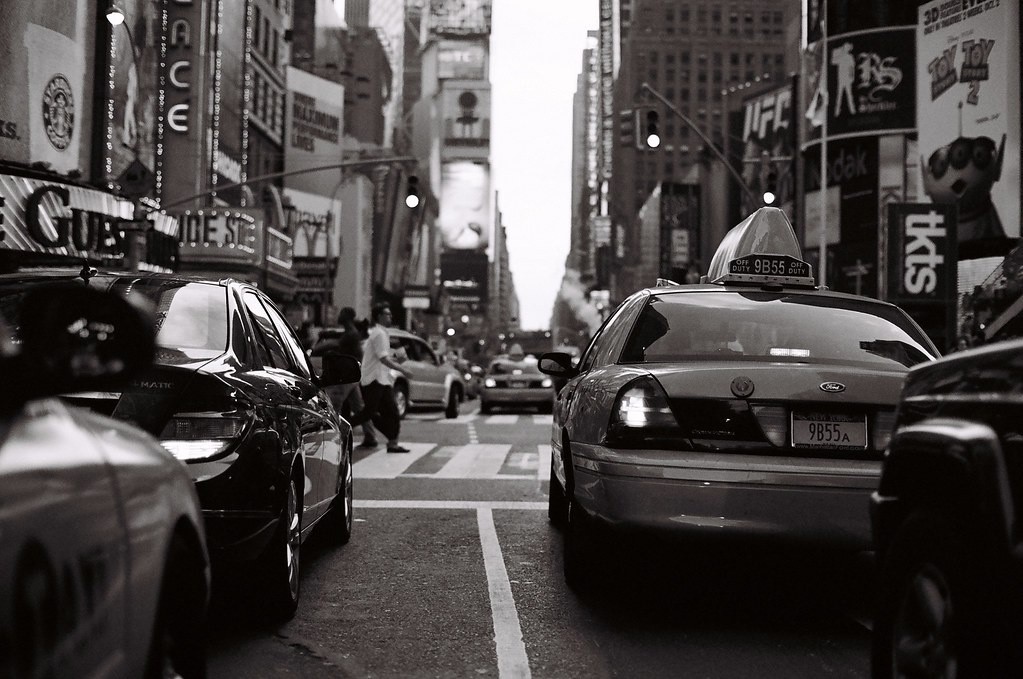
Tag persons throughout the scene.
[326,308,379,450]
[357,303,414,453]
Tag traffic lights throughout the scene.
[404,173,419,209]
[644,110,662,149]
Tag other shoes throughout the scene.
[387,447,410,453]
[356,440,378,449]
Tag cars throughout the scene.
[308,325,467,420]
[0,322,213,679]
[478,354,553,412]
[445,345,485,401]
[865,252,1023,679]
[550,207,944,602]
[0,266,357,622]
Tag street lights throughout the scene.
[105,3,151,265]
